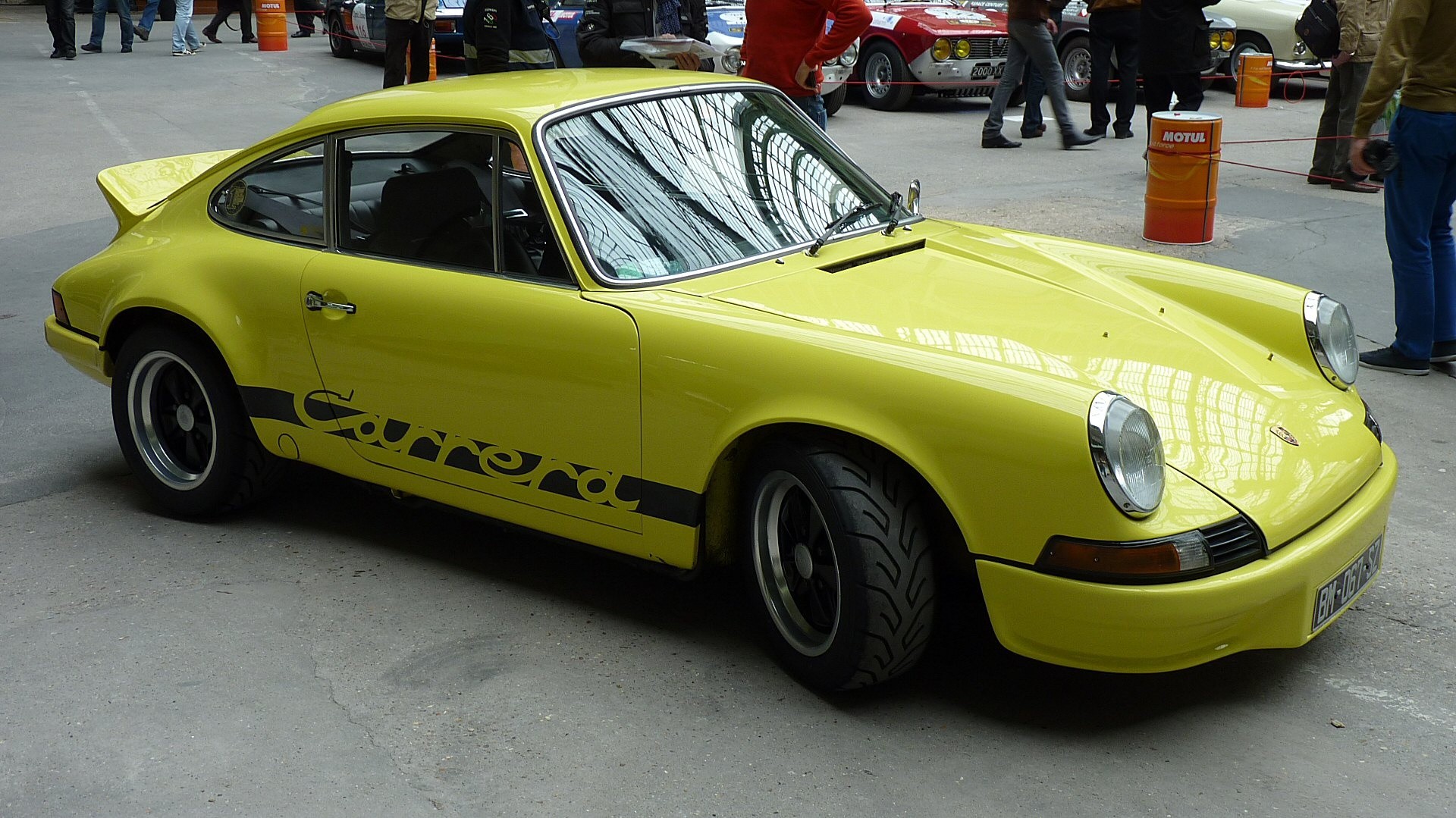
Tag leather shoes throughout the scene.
[1332,175,1380,192]
[1064,134,1105,148]
[291,31,311,38]
[242,37,258,43]
[203,28,222,43]
[1307,169,1334,184]
[982,135,1023,148]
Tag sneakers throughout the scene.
[1036,124,1047,132]
[193,42,206,52]
[81,43,102,53]
[1116,130,1134,139]
[121,45,132,52]
[1430,341,1456,362]
[1356,345,1431,376]
[172,49,196,56]
[133,25,148,41]
[1084,128,1106,138]
[51,48,66,58]
[1022,129,1044,138]
[65,50,77,58]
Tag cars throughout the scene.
[41,64,1396,716]
[325,0,1336,119]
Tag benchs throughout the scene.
[218,165,539,240]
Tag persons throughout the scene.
[44,0,1218,148]
[1306,0,1394,193]
[1347,0,1456,376]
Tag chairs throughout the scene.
[366,165,539,274]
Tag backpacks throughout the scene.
[1295,0,1340,60]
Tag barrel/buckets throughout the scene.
[254,1,290,52]
[406,35,435,84]
[1142,111,1223,244]
[1235,50,1274,108]
